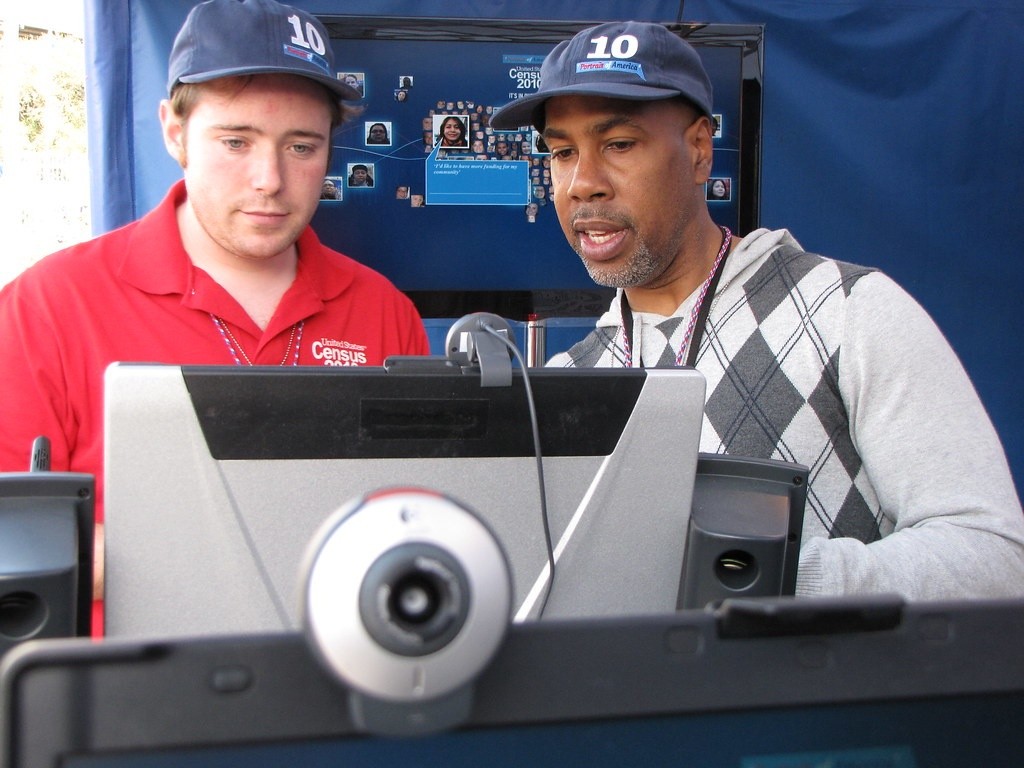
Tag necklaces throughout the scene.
[620,225,734,373]
[207,313,304,366]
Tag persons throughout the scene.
[487,16,1023,603]
[321,179,341,200]
[393,75,414,102]
[2,1,434,648]
[341,73,363,97]
[348,164,373,187]
[705,179,729,200]
[396,186,426,208]
[423,100,554,224]
[367,124,391,145]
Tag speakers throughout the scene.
[0,472,95,651]
[679,454,807,606]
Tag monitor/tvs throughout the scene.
[103,360,708,639]
[0,599,1022,767]
[309,18,767,317]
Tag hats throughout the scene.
[488,20,720,138]
[167,0,362,102]
